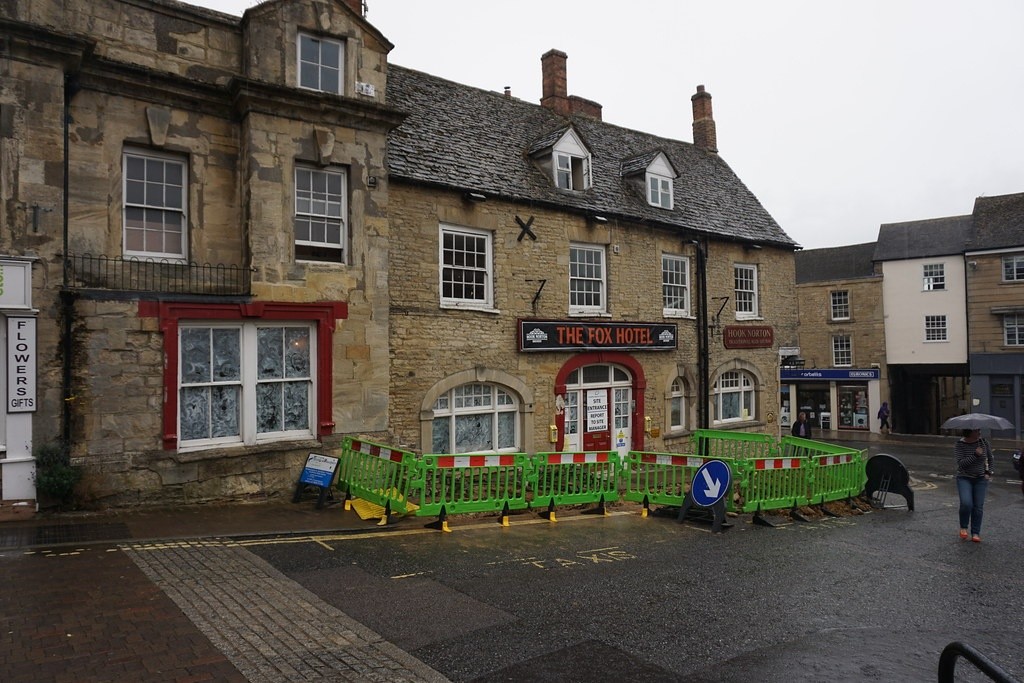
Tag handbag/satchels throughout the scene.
[979,437,990,480]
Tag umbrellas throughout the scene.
[941,413,1017,448]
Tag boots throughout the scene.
[881,429,884,434]
[887,428,892,435]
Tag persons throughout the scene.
[954,427,995,542]
[878,401,894,435]
[791,413,816,464]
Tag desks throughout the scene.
[820,412,831,429]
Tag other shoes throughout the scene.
[972,533,981,542]
[959,529,968,539]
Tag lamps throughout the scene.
[462,192,487,204]
[743,245,762,251]
[586,216,608,225]
[681,239,698,246]
[968,262,977,270]
